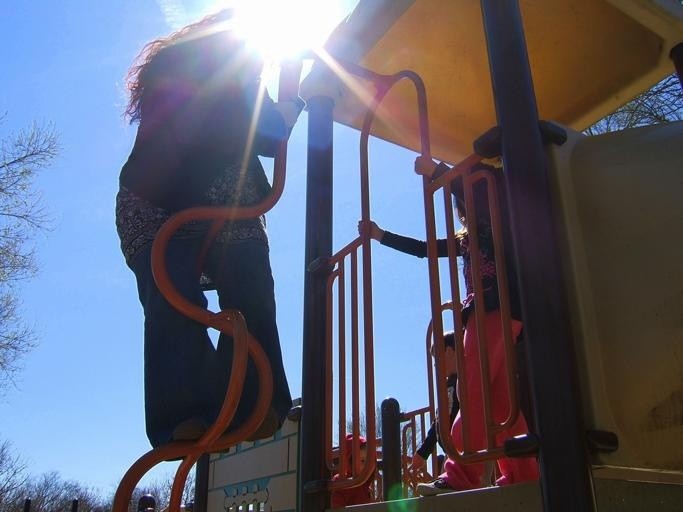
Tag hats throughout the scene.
[346,434,367,457]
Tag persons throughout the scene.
[411,332,459,471]
[330,433,375,509]
[116,6,293,461]
[358,157,541,497]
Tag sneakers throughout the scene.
[214,405,280,441]
[417,477,456,496]
[172,417,229,454]
